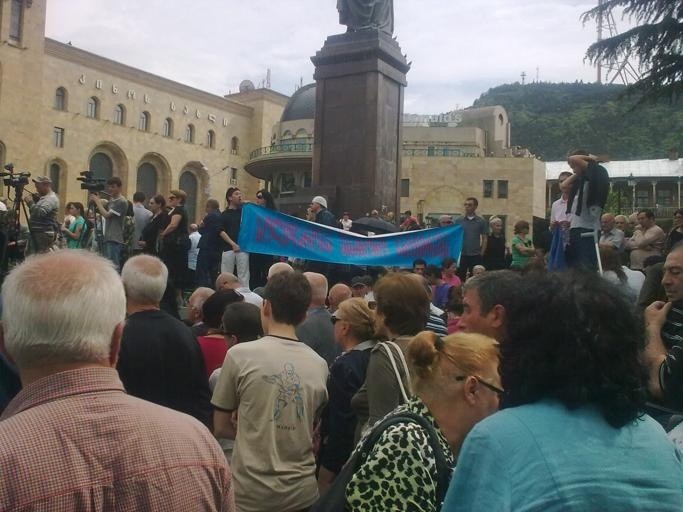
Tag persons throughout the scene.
[302,195,452,286]
[452,146,683,299]
[1,177,277,300]
[1,247,683,511]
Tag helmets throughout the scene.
[311,195,328,208]
[31,175,53,184]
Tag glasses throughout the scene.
[330,315,343,325]
[455,375,511,401]
[368,300,378,310]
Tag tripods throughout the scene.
[74,196,110,255]
[5,196,41,256]
